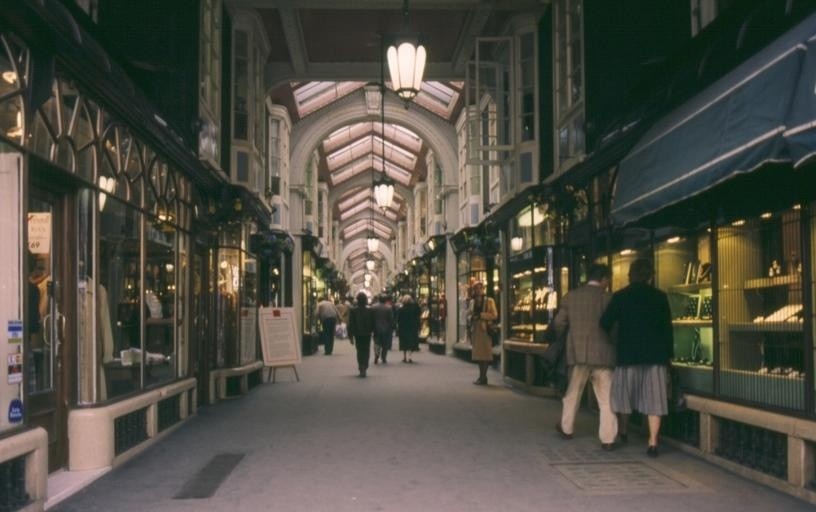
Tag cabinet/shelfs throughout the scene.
[451,183,590,398]
[570,184,816,498]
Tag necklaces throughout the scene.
[691,340,701,360]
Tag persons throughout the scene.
[463,281,498,386]
[313,282,428,379]
[600,258,675,456]
[550,261,619,451]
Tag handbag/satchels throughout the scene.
[486,323,497,336]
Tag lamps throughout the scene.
[360,1,428,289]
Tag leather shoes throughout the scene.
[555,421,573,439]
[601,441,616,451]
[474,377,486,384]
[646,446,656,456]
[620,431,628,443]
